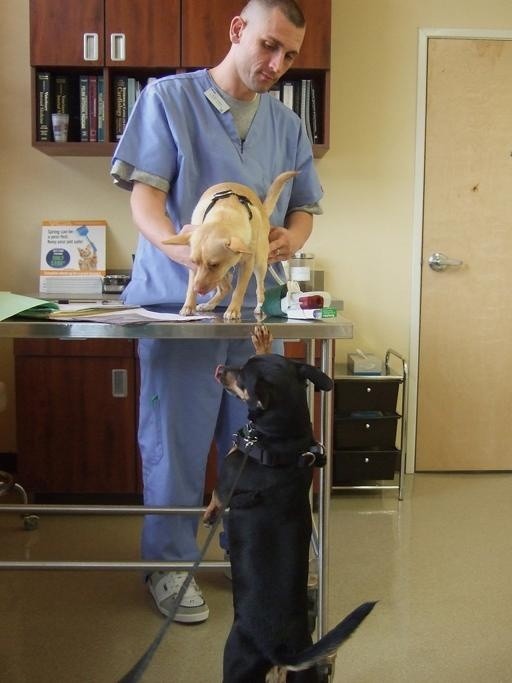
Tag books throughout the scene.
[37,72,323,143]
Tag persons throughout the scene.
[110,0,324,624]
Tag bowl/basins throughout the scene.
[102,274,131,293]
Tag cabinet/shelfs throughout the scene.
[13,337,334,504]
[331,347,409,501]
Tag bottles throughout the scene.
[285,253,316,291]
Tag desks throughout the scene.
[0,306,352,683]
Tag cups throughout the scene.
[260,283,288,316]
[52,113,70,141]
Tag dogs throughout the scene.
[200,323,378,682]
[162,167,302,320]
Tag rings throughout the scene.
[275,249,280,256]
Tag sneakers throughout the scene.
[146,568,209,624]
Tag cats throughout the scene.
[78,244,98,271]
